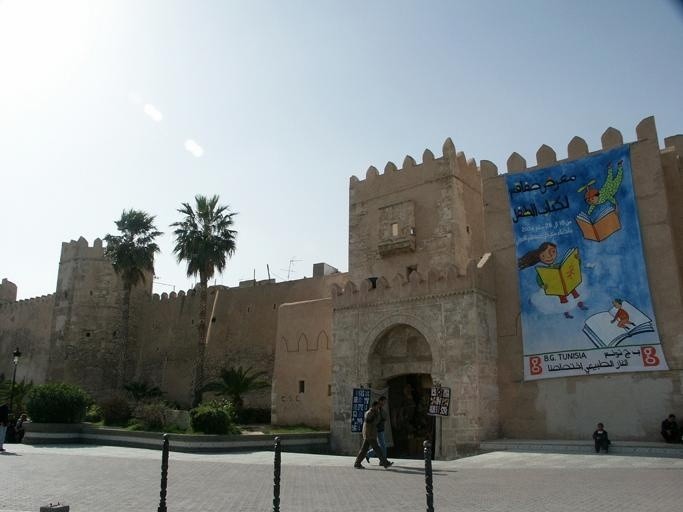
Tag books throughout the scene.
[575,206,622,242]
[534,247,582,296]
[582,300,655,349]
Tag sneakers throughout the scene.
[354,463,365,469]
[383,461,394,469]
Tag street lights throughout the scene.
[9,348,21,413]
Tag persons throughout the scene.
[517,242,590,319]
[660,414,680,444]
[0,399,11,451]
[364,396,391,466]
[583,159,624,218]
[10,413,26,443]
[591,423,610,453]
[609,299,638,330]
[353,401,393,469]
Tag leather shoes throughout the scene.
[365,457,370,464]
